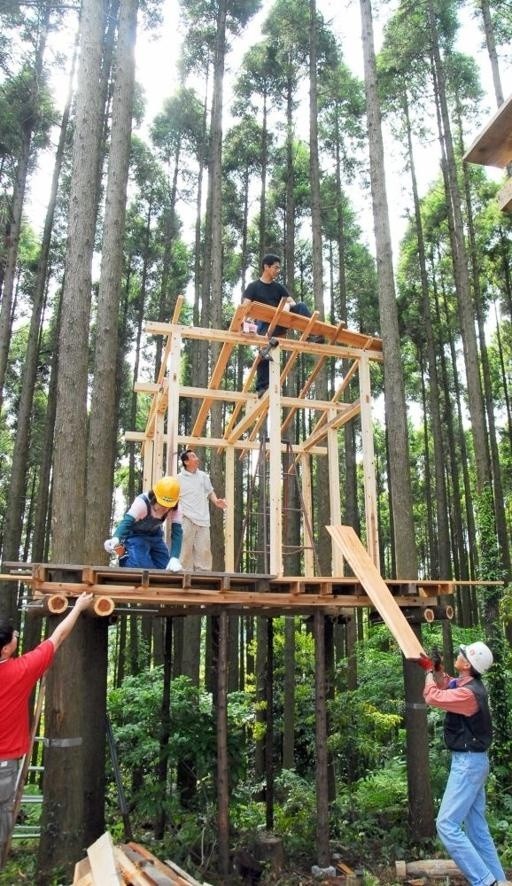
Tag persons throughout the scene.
[0,592,94,865]
[415,641,507,886]
[171,504,182,557]
[176,448,228,572]
[238,253,325,398]
[103,477,181,569]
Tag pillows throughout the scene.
[459,641,494,674]
[152,476,181,508]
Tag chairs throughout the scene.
[119,554,128,567]
[306,334,324,343]
[258,386,266,398]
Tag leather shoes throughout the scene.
[165,557,182,573]
[413,653,433,671]
[429,645,441,671]
[104,537,120,554]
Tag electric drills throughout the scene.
[258,338,280,364]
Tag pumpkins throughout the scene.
[234,432,323,576]
[3,672,133,860]
